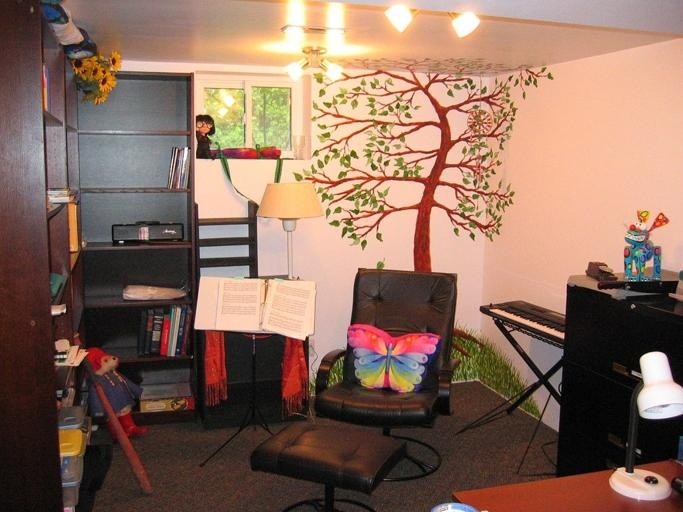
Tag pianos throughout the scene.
[480,299,567,348]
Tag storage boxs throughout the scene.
[56,407,93,512]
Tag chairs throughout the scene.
[314,268,458,482]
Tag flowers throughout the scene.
[71,51,122,105]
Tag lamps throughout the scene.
[446,9,480,38]
[609,350,683,501]
[255,183,324,280]
[385,2,421,33]
[324,0,346,31]
[284,0,305,28]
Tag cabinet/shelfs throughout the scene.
[555,266,683,476]
[77,73,198,424]
[0,0,92,512]
[196,274,309,430]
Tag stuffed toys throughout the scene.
[83,344,151,443]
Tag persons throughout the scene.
[192,112,218,160]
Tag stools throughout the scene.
[251,419,407,512]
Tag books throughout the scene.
[135,274,317,359]
[54,337,90,369]
[44,185,81,256]
[163,145,191,192]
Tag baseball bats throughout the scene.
[72,334,153,495]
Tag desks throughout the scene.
[451,458,683,512]
